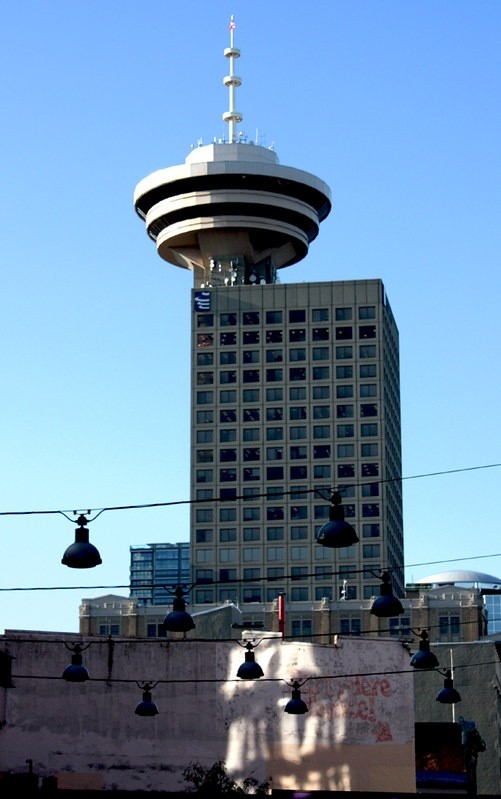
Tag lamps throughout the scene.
[61,488,461,715]
